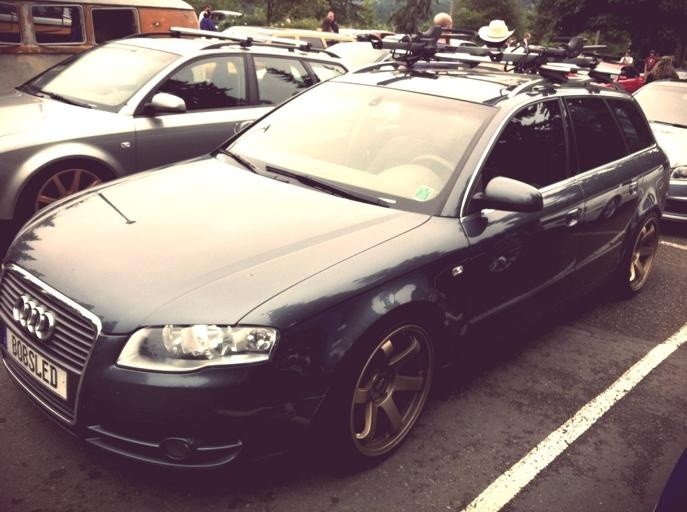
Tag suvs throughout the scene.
[0,25,351,233]
[1,27,675,491]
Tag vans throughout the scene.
[0,0,203,99]
[210,9,245,31]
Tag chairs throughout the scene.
[174,69,192,84]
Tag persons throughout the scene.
[644,50,657,73]
[619,49,633,65]
[520,33,531,52]
[647,56,679,84]
[317,11,339,33]
[199,4,211,24]
[477,20,515,48]
[200,11,217,31]
[433,13,453,35]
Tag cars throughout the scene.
[593,63,647,94]
[219,25,412,72]
[630,75,687,223]
